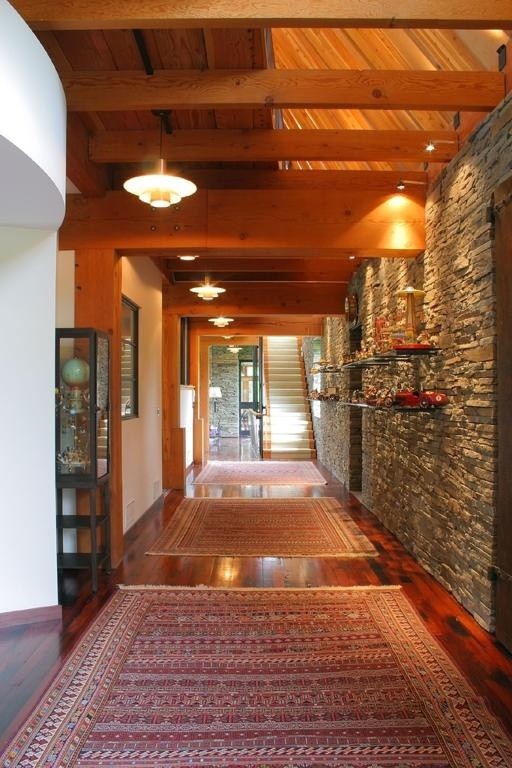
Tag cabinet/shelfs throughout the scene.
[55,327,113,593]
[338,344,441,415]
[304,369,341,402]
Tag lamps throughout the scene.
[189,271,236,329]
[122,109,199,210]
[208,387,223,413]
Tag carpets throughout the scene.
[1,580,511,766]
[193,460,326,485]
[144,497,379,558]
